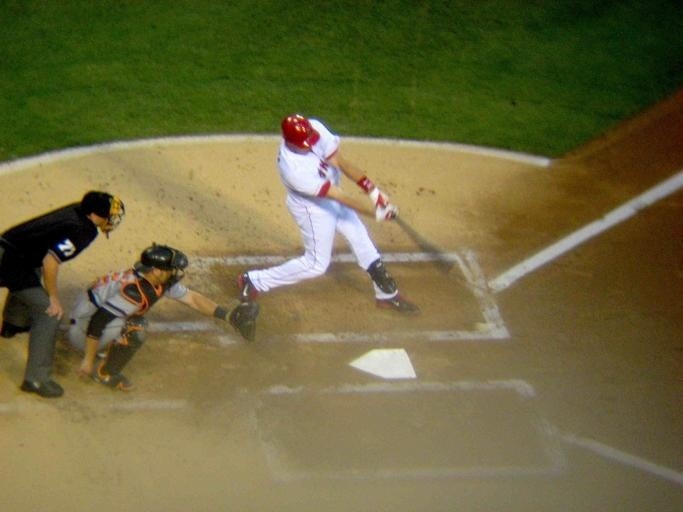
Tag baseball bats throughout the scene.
[381,205,455,276]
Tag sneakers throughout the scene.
[1,323,32,338]
[20,377,64,397]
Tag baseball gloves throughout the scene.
[230,301,259,342]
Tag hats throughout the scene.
[282,114,320,149]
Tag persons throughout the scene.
[236,112,420,315]
[67,245,260,392]
[0,191,125,399]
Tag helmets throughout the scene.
[133,244,188,273]
[82,192,115,218]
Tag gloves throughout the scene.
[369,187,399,222]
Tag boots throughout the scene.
[237,272,259,303]
[376,292,421,317]
[94,369,137,389]
